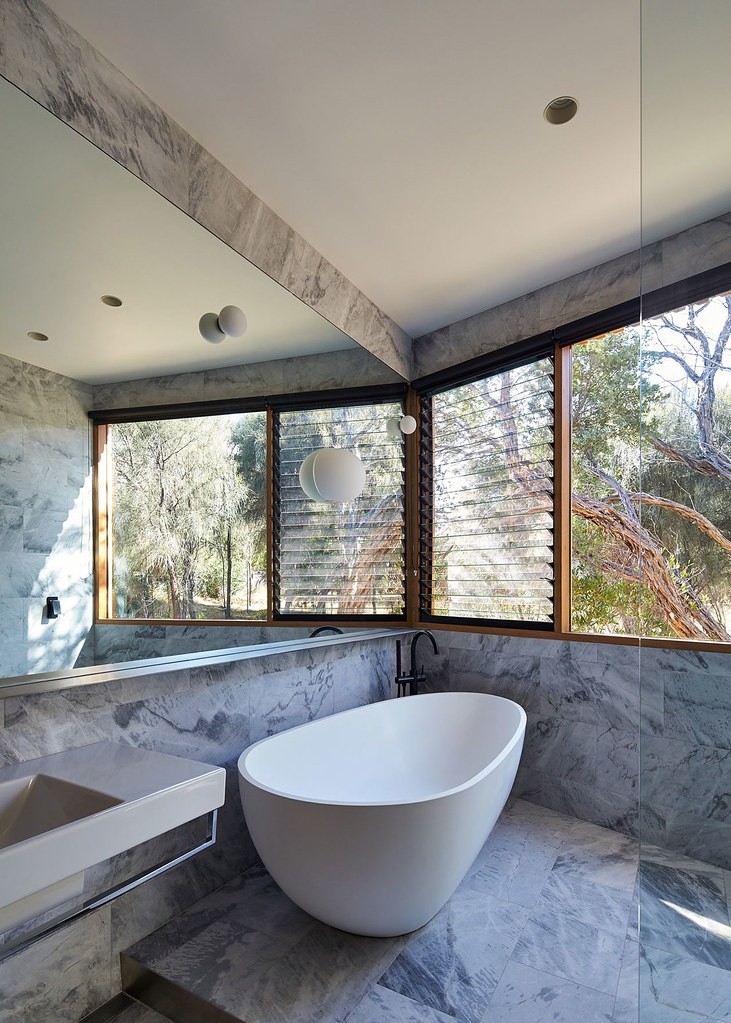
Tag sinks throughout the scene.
[1,772,131,902]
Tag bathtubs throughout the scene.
[236,692,527,938]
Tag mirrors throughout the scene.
[0,71,414,698]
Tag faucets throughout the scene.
[309,625,344,638]
[395,629,440,697]
